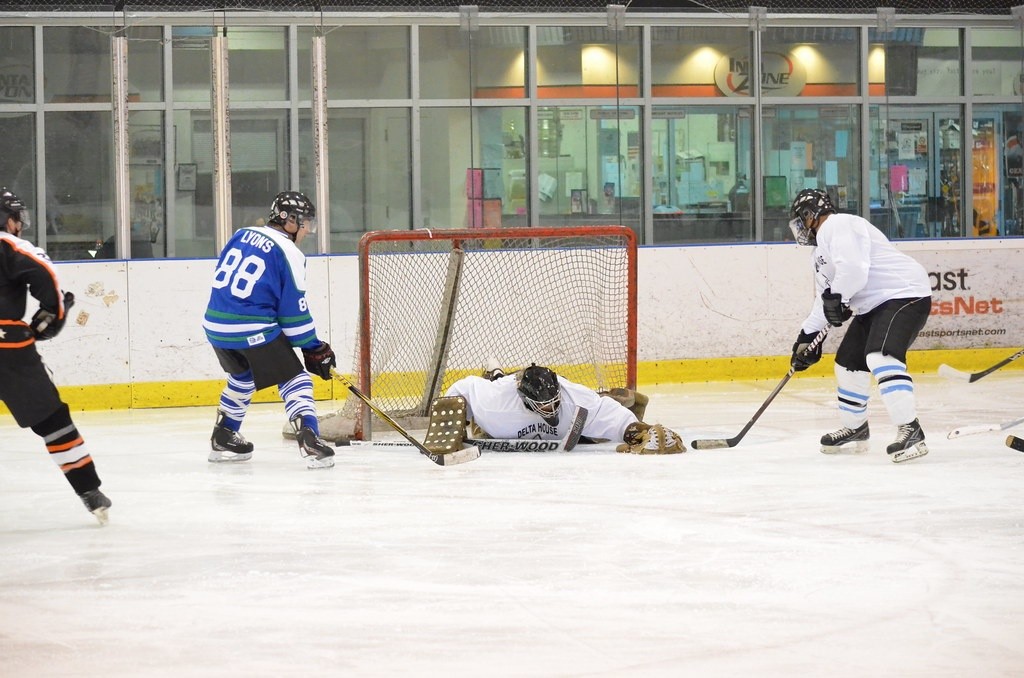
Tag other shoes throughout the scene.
[493,368,504,376]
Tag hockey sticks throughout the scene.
[334,405,588,452]
[326,364,482,467]
[1006,435,1024,452]
[935,349,1024,384]
[37,315,52,334]
[947,418,1024,442]
[691,302,850,451]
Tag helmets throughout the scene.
[788,188,837,246]
[269,191,315,226]
[0,187,27,230]
[516,364,562,428]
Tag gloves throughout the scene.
[820,287,853,327]
[301,340,336,381]
[790,327,827,372]
[30,290,75,340]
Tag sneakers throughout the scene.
[886,417,929,463]
[207,407,254,462]
[820,420,871,454]
[80,486,113,526]
[290,413,336,469]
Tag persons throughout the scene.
[0,189,112,527]
[444,358,689,455]
[789,188,932,463]
[202,192,338,469]
